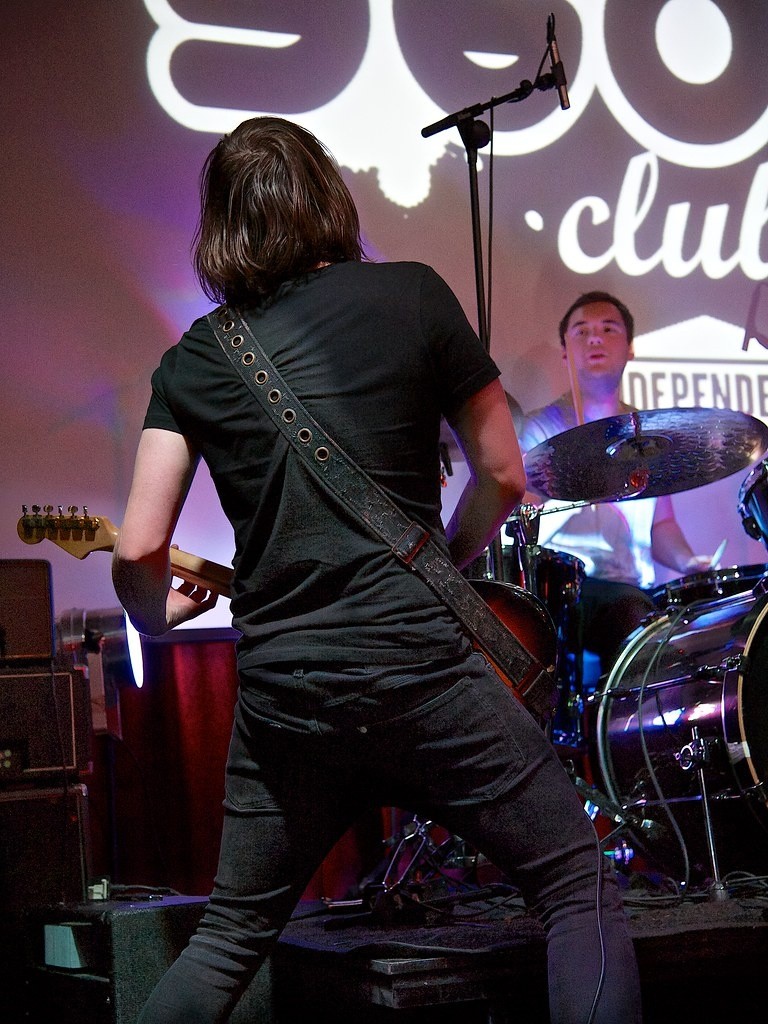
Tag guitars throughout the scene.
[14,502,558,732]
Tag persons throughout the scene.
[517,292,721,588]
[112,117,640,1024]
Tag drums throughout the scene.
[458,545,586,756]
[581,578,660,747]
[597,577,768,883]
[736,457,768,555]
[648,562,768,611]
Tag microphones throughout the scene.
[546,18,570,110]
[743,284,760,351]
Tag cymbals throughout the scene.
[521,406,768,504]
[440,389,533,462]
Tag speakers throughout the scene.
[22,895,273,1024]
[0,558,57,663]
[0,785,91,928]
[0,664,94,785]
